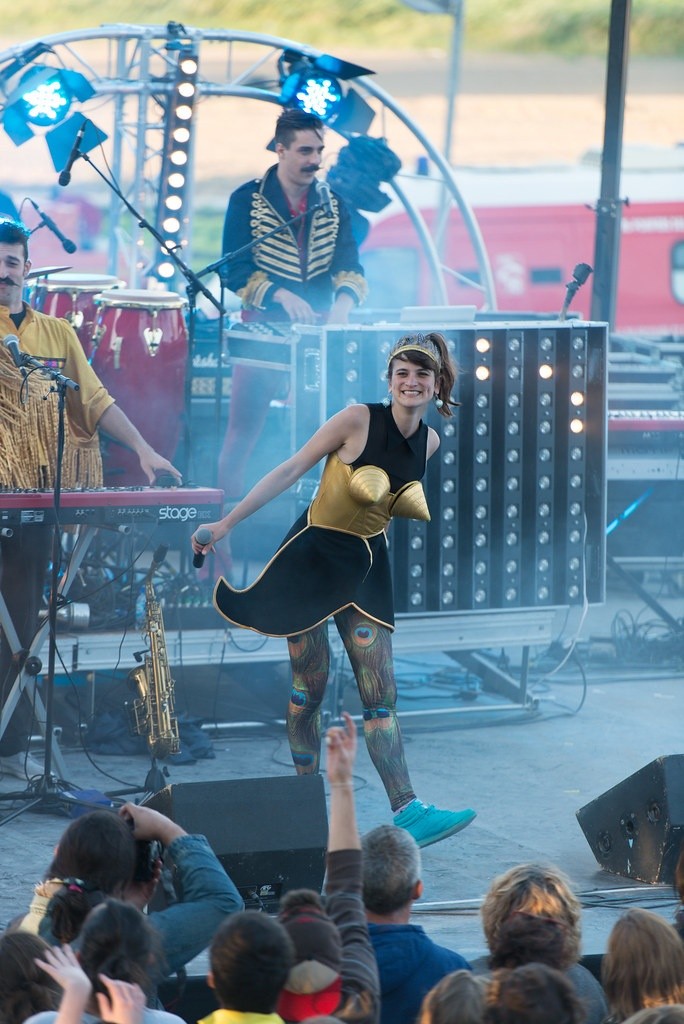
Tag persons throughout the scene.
[191,331,479,854]
[0,710,684,1024]
[0,220,181,779]
[216,109,366,579]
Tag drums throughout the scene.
[86,287,190,485]
[29,272,125,359]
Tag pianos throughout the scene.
[0,485,228,785]
[605,408,683,633]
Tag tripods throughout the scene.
[0,354,121,826]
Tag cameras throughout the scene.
[126,820,160,881]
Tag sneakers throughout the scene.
[0,752,55,781]
[393,799,476,848]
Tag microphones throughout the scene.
[192,528,212,568]
[29,200,77,254]
[3,334,27,379]
[59,122,86,186]
[315,181,334,219]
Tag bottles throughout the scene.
[135,585,149,631]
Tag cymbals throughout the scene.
[26,265,73,281]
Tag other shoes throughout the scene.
[198,550,233,583]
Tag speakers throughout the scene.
[138,774,328,915]
[576,754,684,885]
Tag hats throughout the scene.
[274,906,345,1020]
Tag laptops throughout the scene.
[400,305,476,324]
